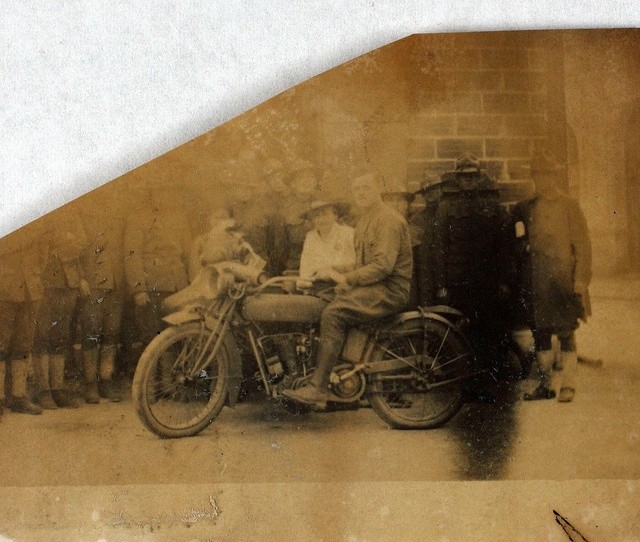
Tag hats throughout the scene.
[448,153,485,173]
[521,148,565,172]
[298,190,351,219]
[380,185,415,202]
[414,171,446,195]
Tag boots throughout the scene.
[282,351,337,404]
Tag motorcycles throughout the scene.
[130,226,471,439]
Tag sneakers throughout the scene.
[0,400,5,414]
[52,390,78,406]
[85,383,98,402]
[99,380,120,401]
[39,390,57,409]
[524,383,556,399]
[10,398,42,414]
[557,387,576,401]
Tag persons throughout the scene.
[1,223,44,416]
[78,232,124,405]
[123,182,205,403]
[508,145,592,405]
[114,300,140,381]
[271,157,335,413]
[31,197,85,413]
[411,169,445,385]
[522,310,562,371]
[60,310,86,399]
[186,204,271,404]
[282,166,414,411]
[295,192,362,410]
[380,176,419,409]
